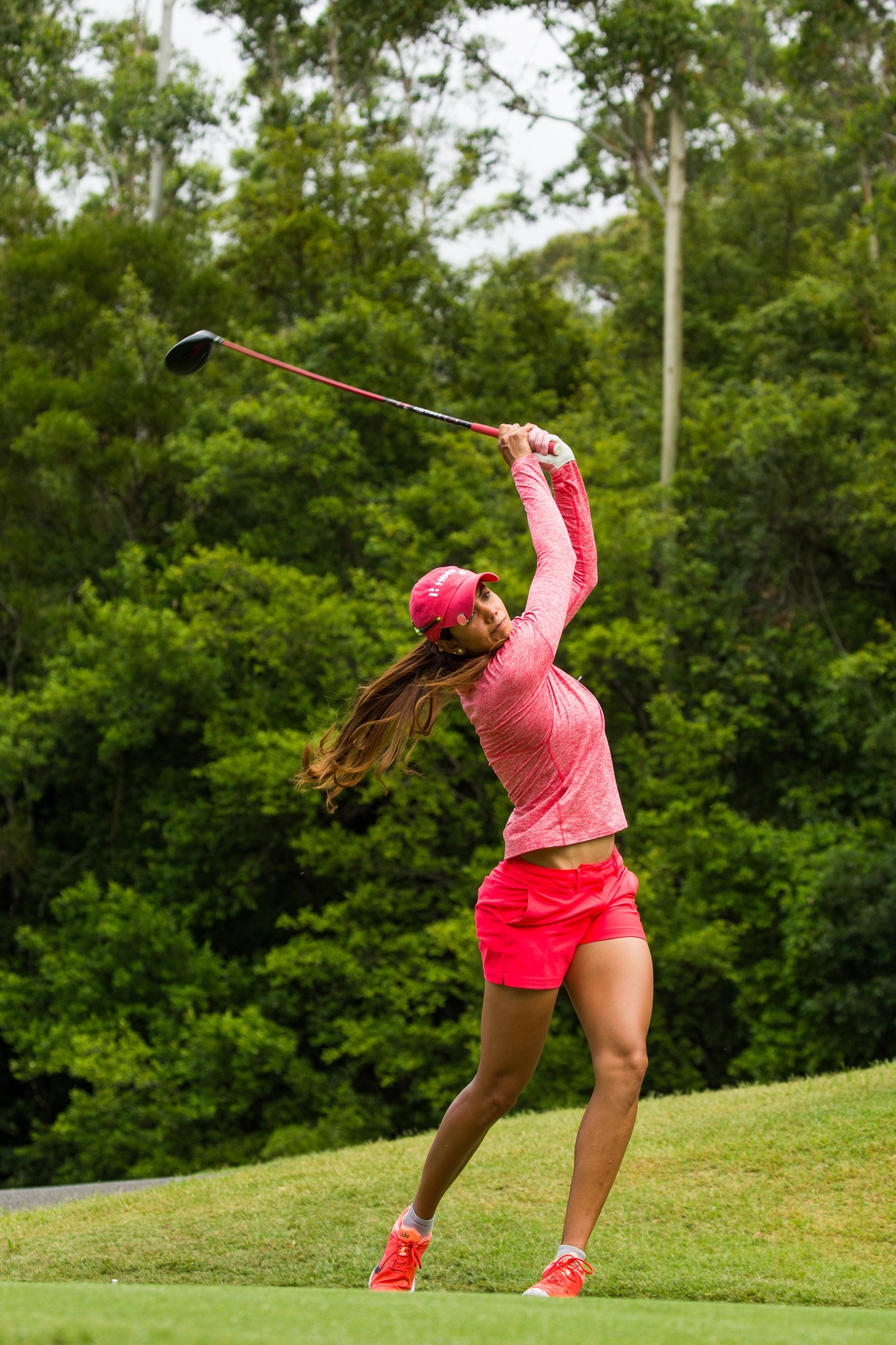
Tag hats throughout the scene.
[410,566,499,645]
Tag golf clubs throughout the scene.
[163,330,563,458]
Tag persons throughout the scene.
[290,423,654,1297]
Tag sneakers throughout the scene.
[522,1255,593,1299]
[368,1206,432,1291]
